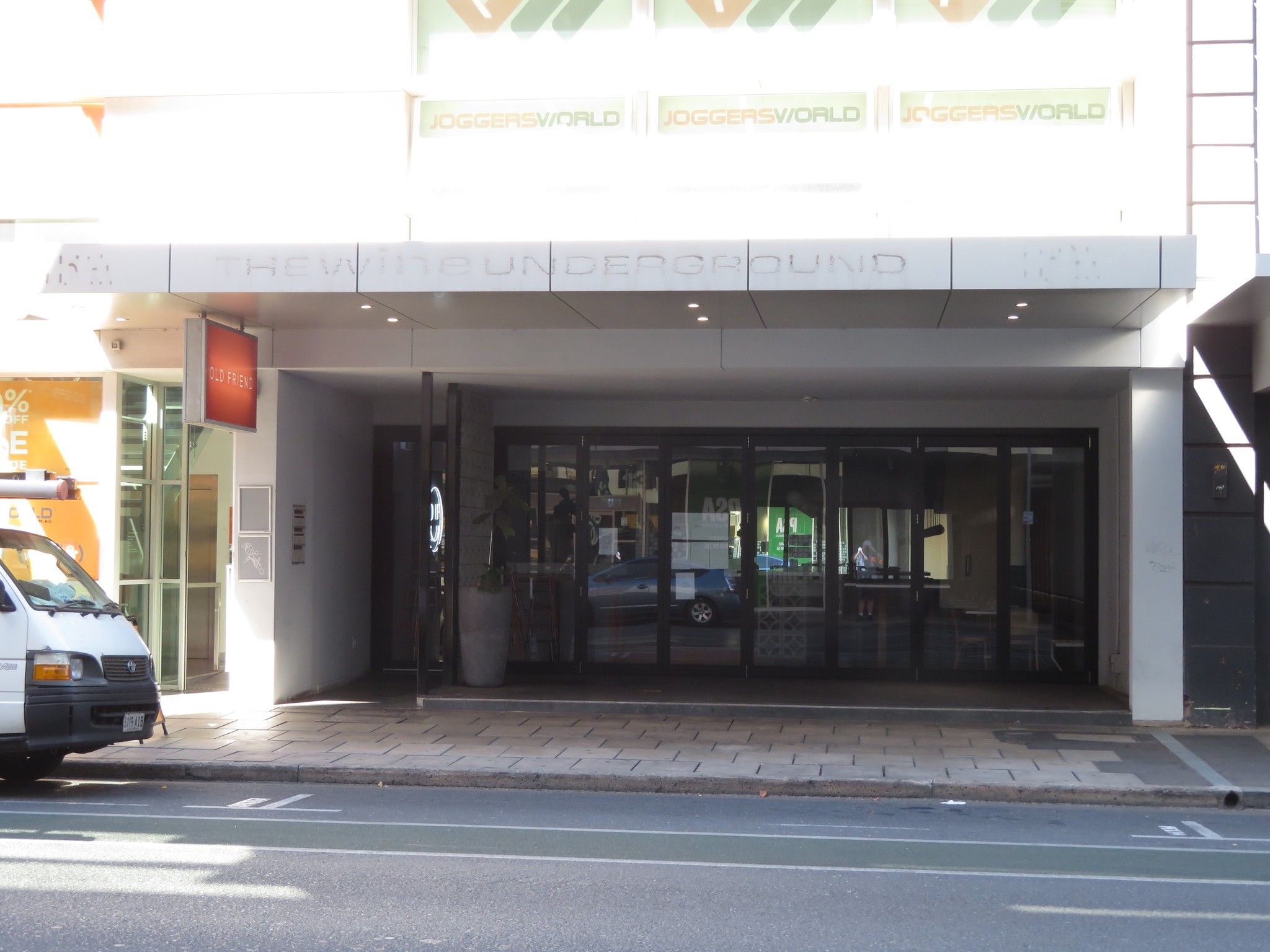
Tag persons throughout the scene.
[852,541,881,622]
[854,548,868,579]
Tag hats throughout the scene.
[863,541,872,546]
[858,547,862,551]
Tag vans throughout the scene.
[0,499,161,782]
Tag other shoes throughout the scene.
[867,614,874,621]
[856,615,864,622]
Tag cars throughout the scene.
[756,553,791,570]
[587,555,740,628]
